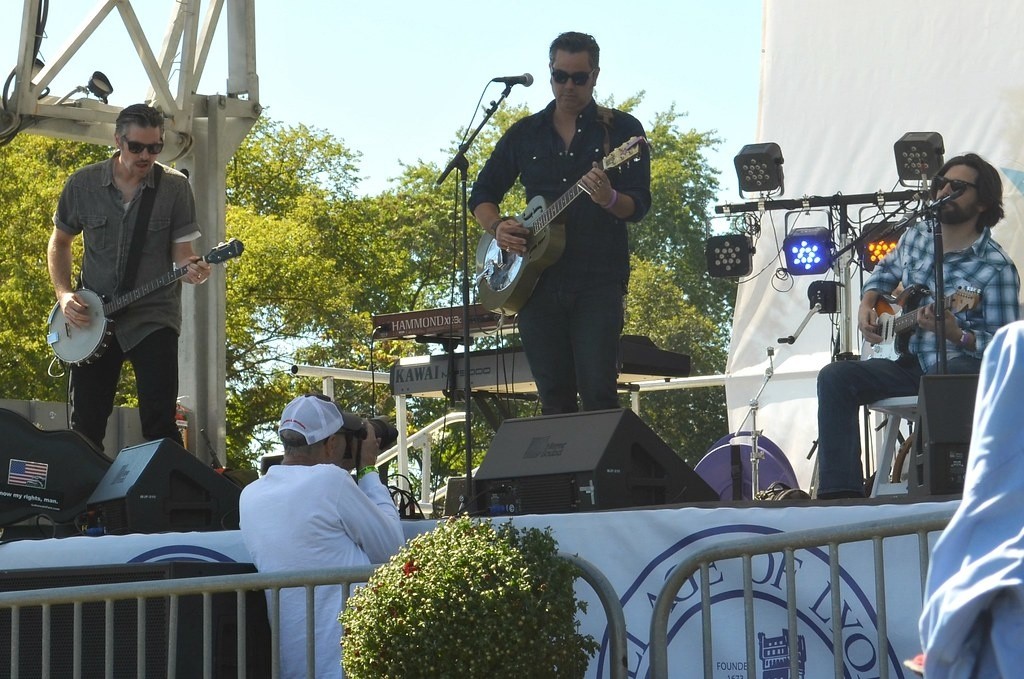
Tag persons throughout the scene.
[47,102,210,448]
[468,32,651,416]
[816,150,1021,497]
[239,393,406,679]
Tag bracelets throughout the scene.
[359,466,378,479]
[956,330,968,347]
[601,189,617,210]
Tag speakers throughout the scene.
[907,374,981,496]
[85,439,242,534]
[442,408,721,516]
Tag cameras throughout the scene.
[353,415,398,450]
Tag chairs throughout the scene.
[865,343,917,497]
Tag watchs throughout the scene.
[489,219,503,236]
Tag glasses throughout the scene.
[122,135,164,154]
[324,431,352,444]
[549,65,595,85]
[935,174,978,193]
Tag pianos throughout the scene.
[374,302,690,434]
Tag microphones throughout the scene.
[874,214,916,242]
[492,72,534,86]
[920,159,930,206]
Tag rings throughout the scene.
[197,273,202,279]
[506,247,510,252]
[597,180,604,187]
[592,184,599,191]
[578,182,593,195]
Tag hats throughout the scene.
[277,392,361,446]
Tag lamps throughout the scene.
[89,72,114,104]
[704,131,944,277]
[31,58,45,81]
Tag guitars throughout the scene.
[859,282,981,368]
[474,134,651,318]
[46,236,245,368]
